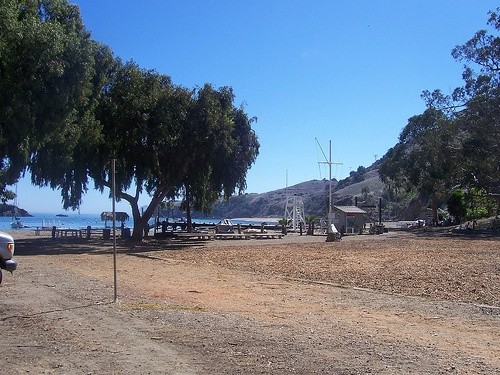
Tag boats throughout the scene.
[11,221,29,228]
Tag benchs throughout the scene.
[173,232,211,240]
[213,233,252,239]
[253,234,285,238]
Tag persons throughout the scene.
[339,226,345,241]
